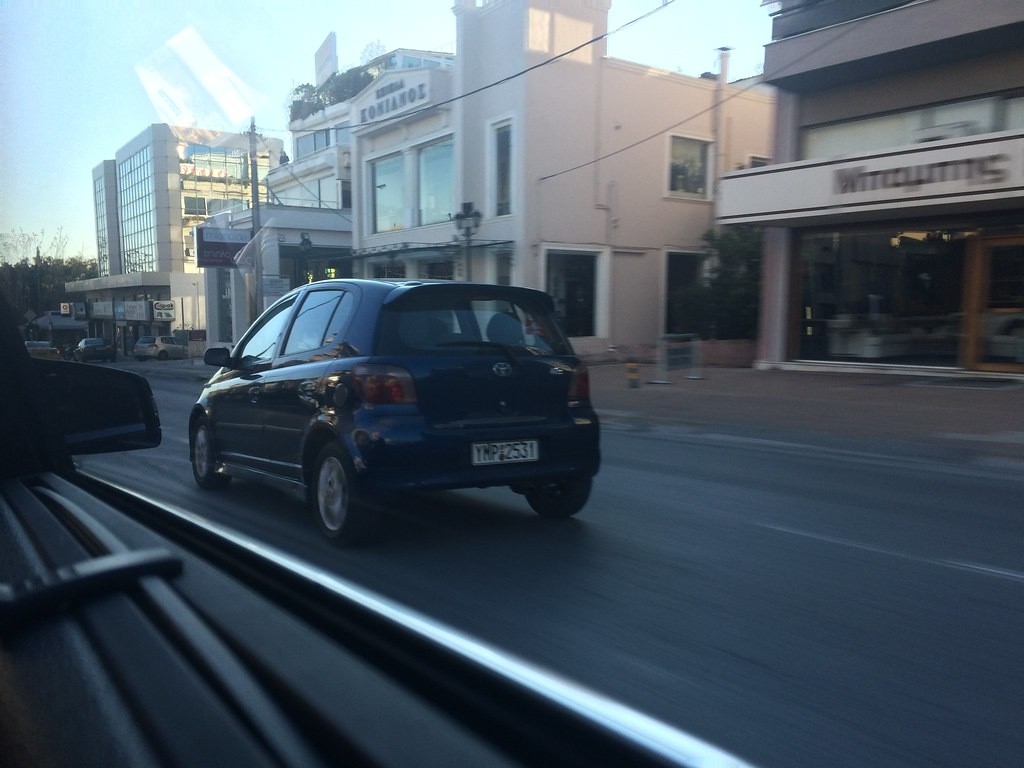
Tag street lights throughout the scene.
[454,203,482,282]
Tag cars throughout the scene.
[74,337,116,363]
[23,340,64,362]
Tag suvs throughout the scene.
[189,277,602,548]
[133,336,188,362]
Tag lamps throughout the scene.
[301,232,312,246]
[889,230,900,249]
[279,152,290,167]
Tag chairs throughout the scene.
[407,307,455,349]
[484,312,523,348]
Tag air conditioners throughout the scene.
[186,248,195,257]
